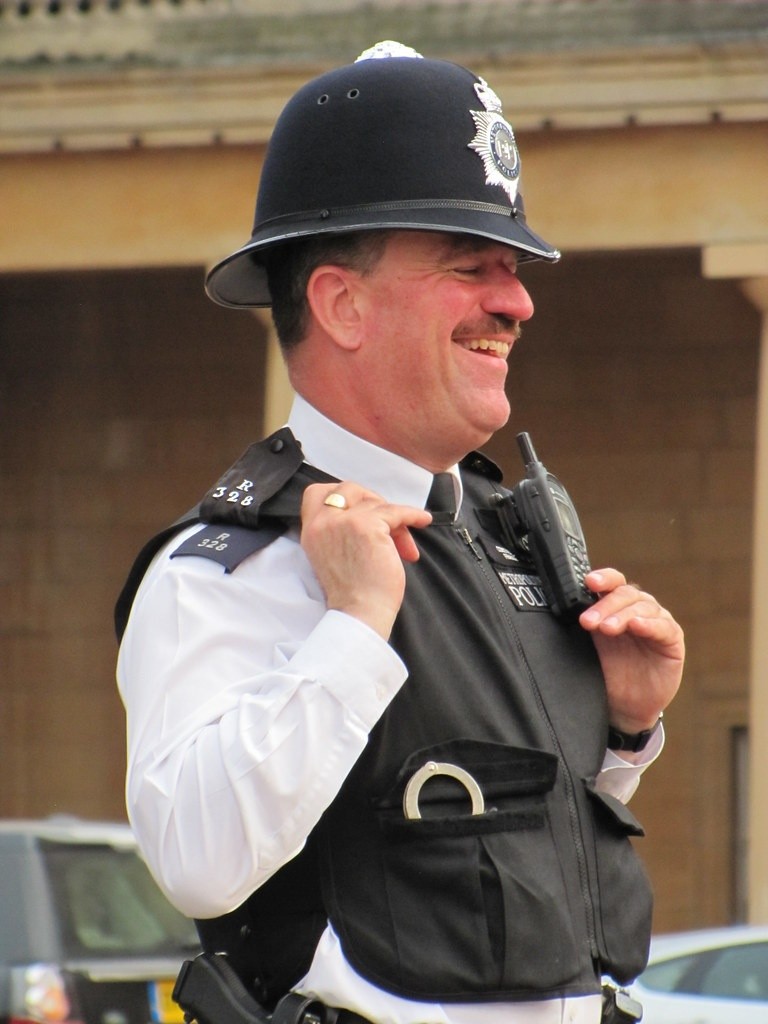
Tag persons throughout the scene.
[116,33,688,1024]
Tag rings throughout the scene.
[323,494,349,510]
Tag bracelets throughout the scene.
[606,712,664,755]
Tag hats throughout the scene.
[206,40,561,310]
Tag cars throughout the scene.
[599,920,768,1024]
[0,812,217,1024]
